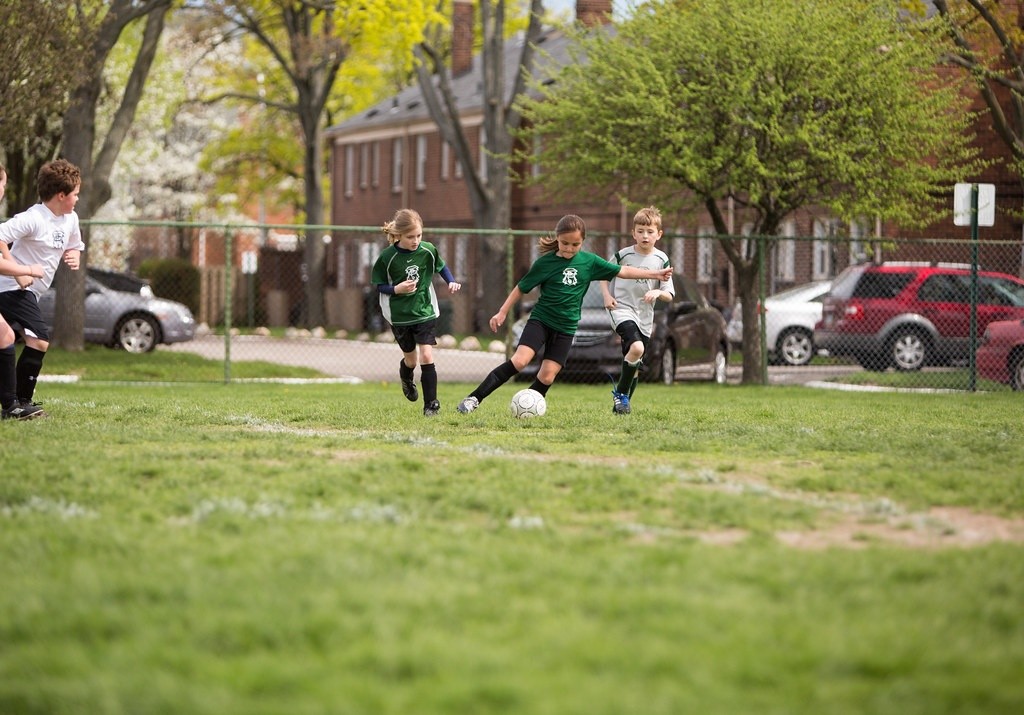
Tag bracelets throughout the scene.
[29,266,32,276]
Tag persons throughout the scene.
[0,164,45,421]
[457,214,673,415]
[600,206,676,416]
[370,208,462,414]
[0,158,85,415]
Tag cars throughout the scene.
[811,260,1024,373]
[37,266,198,357]
[973,319,1024,394]
[726,279,836,368]
[505,269,733,388]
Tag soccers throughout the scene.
[510,388,546,419]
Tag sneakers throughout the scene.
[400,368,418,402]
[423,400,440,416]
[456,396,480,416]
[1,400,44,421]
[605,370,631,414]
[18,401,50,420]
